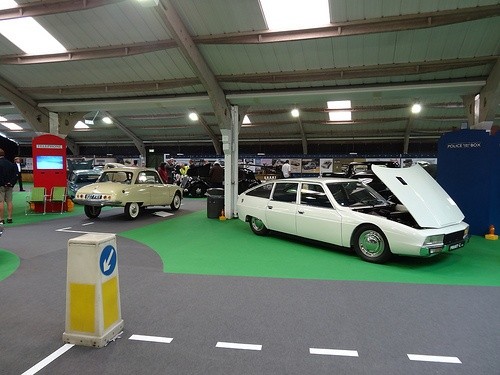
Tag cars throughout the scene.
[68,170,100,199]
[345,161,401,202]
[186,164,259,197]
[74,166,183,219]
[235,163,471,263]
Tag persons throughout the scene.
[158,158,224,188]
[131,160,138,166]
[0,148,25,224]
[281,160,291,178]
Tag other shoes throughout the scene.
[0,220,4,224]
[7,218,12,222]
[20,189,25,191]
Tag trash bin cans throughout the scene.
[205,188,225,219]
